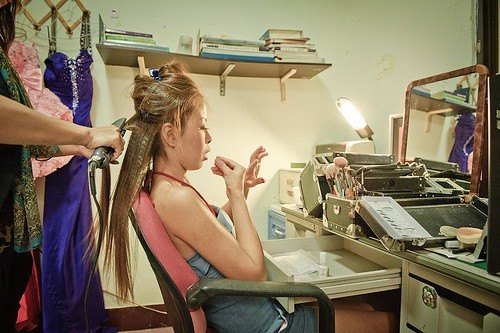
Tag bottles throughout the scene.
[178,35,192,55]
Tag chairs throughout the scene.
[128,188,336,333]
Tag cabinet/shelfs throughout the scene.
[281,203,500,333]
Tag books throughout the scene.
[410,80,471,104]
[197,30,324,62]
[103,28,170,52]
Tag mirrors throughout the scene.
[400,64,490,203]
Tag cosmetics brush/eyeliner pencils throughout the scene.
[325,157,359,201]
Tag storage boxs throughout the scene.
[267,206,285,240]
[323,192,489,254]
[299,152,426,216]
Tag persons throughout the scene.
[82,61,400,333]
[1,0,126,333]
